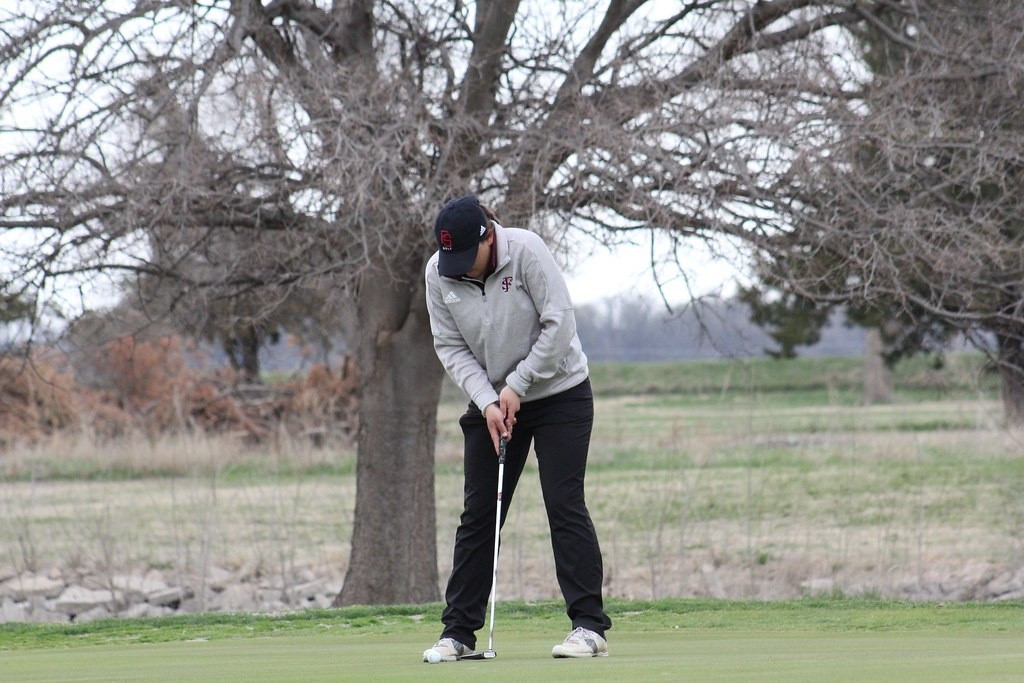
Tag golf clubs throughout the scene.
[460,436,507,659]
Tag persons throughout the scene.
[426,197,613,665]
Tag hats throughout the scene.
[434,196,487,275]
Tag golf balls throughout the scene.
[427,650,442,663]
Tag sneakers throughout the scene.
[422,638,474,662]
[552,627,609,658]
